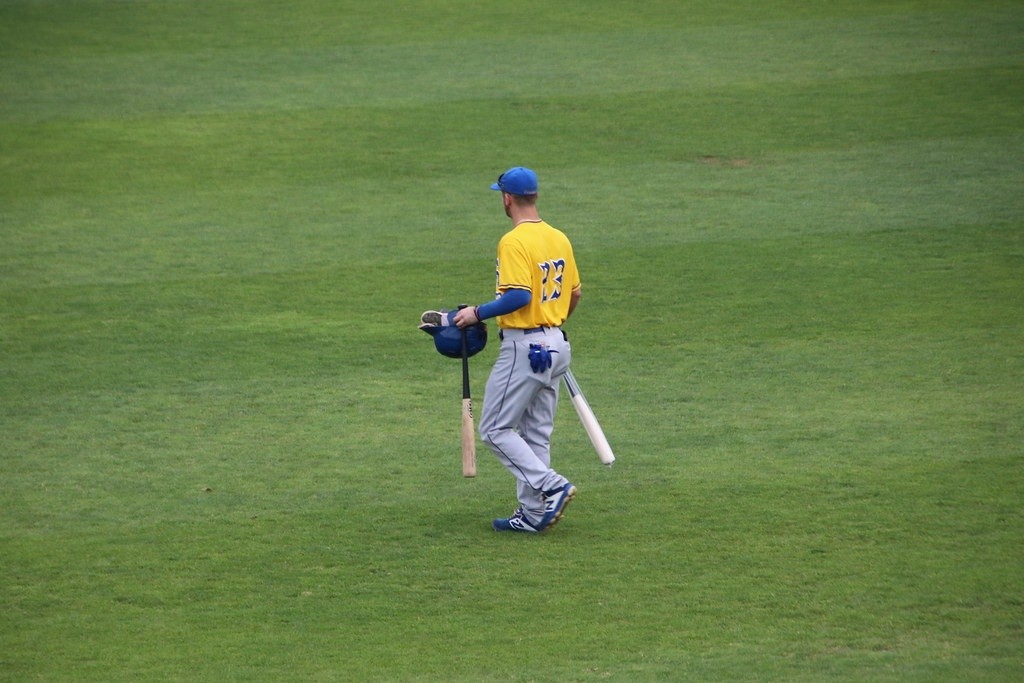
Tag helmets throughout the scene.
[419,313,487,358]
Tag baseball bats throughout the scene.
[561,367,616,466]
[457,304,477,478]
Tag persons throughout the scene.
[453,167,582,531]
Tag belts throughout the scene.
[498,327,543,340]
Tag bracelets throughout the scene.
[474,305,481,320]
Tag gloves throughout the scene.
[528,346,552,374]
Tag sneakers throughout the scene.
[491,508,541,532]
[538,484,577,531]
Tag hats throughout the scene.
[490,167,537,195]
